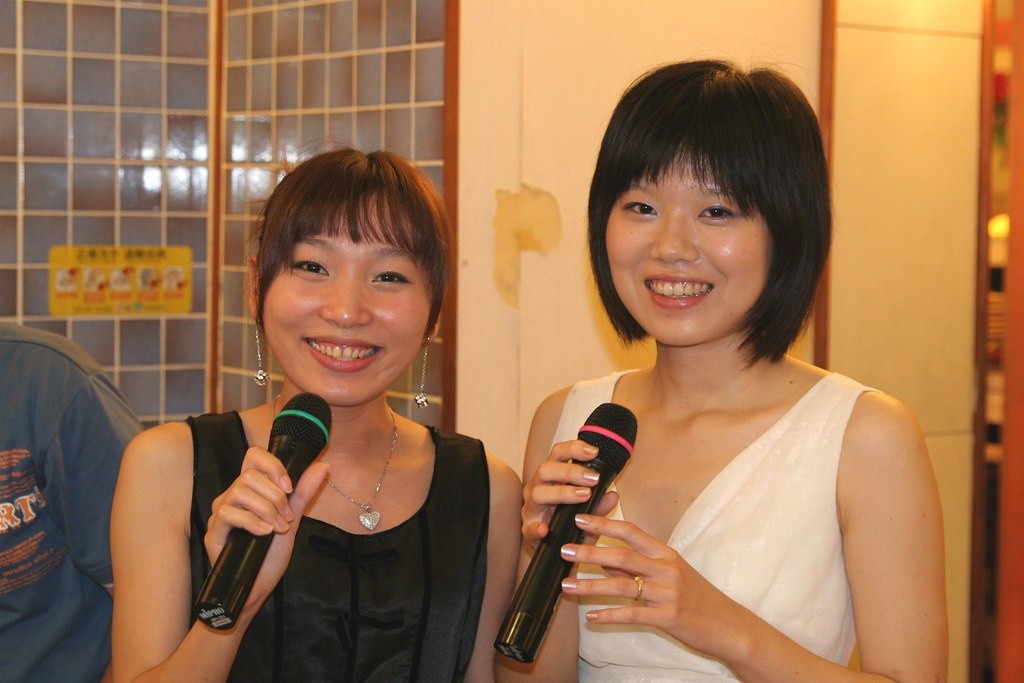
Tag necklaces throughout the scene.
[272,395,398,531]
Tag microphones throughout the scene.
[494,402,637,664]
[194,393,332,630]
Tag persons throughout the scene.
[110,149,522,683]
[496,60,948,683]
[0,322,141,683]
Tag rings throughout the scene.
[634,576,643,601]
